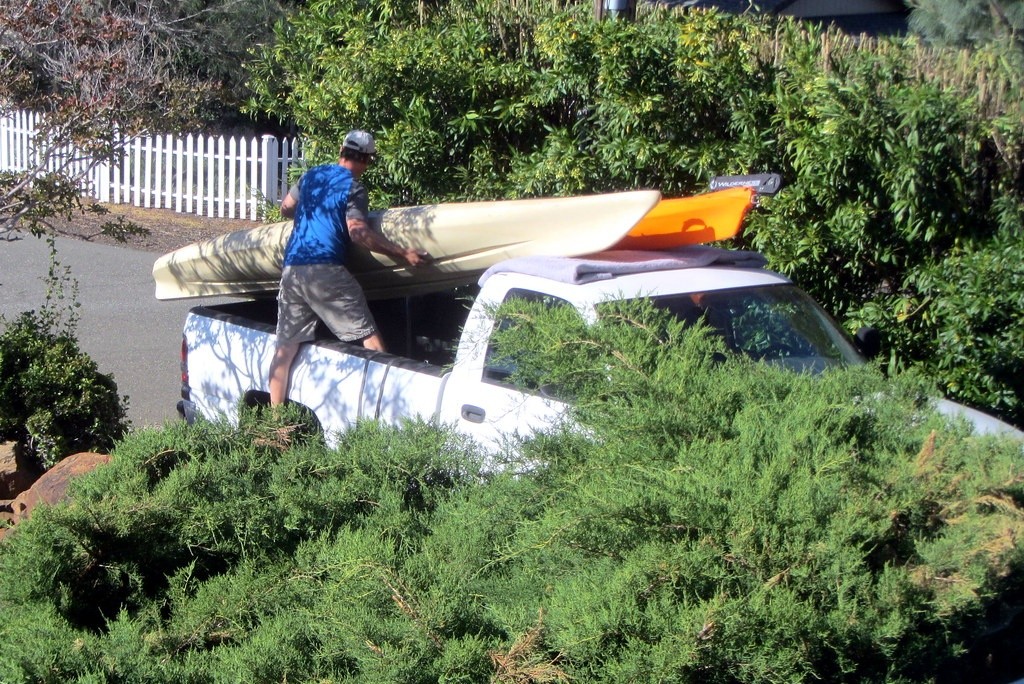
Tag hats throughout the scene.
[342,130,377,153]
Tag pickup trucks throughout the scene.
[177,262,1023,483]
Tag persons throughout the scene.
[268,130,428,422]
